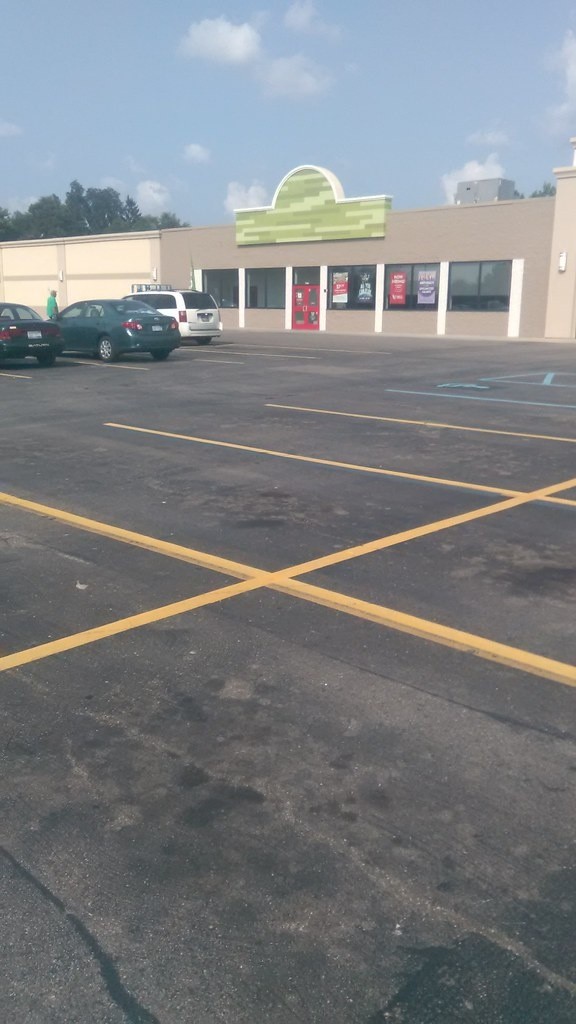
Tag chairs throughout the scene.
[0,316,11,321]
[90,309,100,317]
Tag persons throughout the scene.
[46,290,58,319]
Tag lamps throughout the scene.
[152,267,157,281]
[59,269,63,281]
[557,251,567,272]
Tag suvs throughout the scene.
[100,289,224,346]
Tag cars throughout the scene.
[0,302,65,365]
[45,298,183,363]
